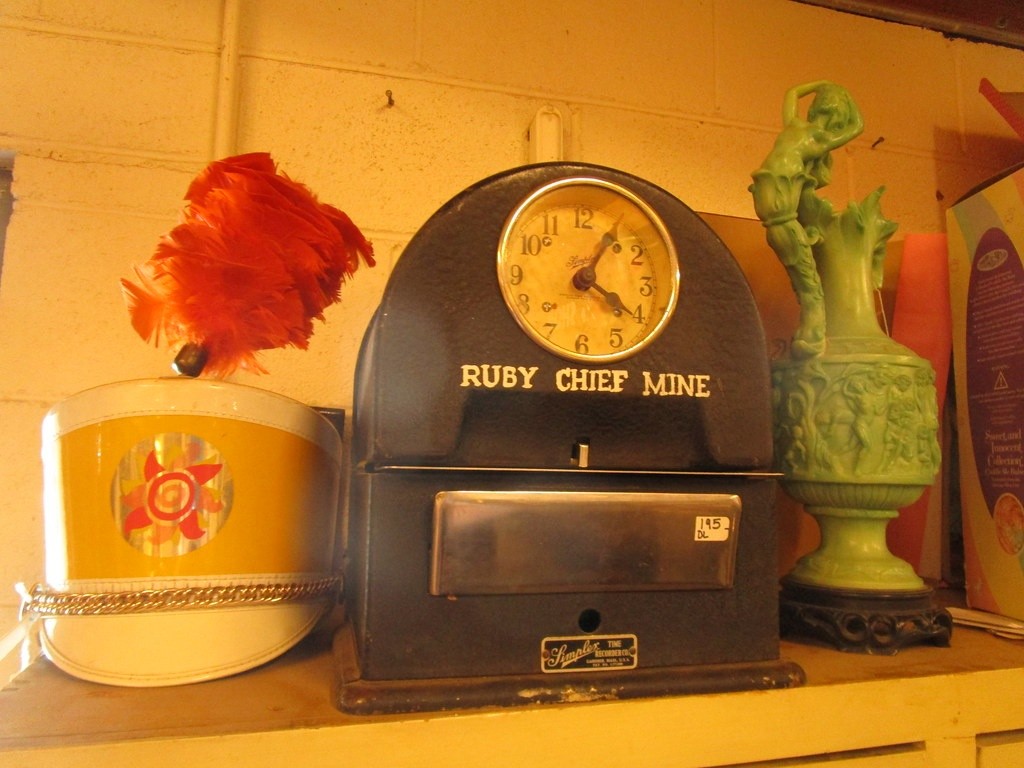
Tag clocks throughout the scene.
[329,158,806,717]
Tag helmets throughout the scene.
[20,151,376,688]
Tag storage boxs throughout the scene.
[945,162,1024,622]
